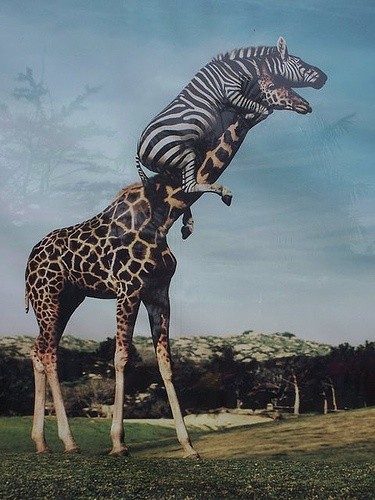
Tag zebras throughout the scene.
[136,35,329,241]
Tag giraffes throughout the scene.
[23,62,313,462]
